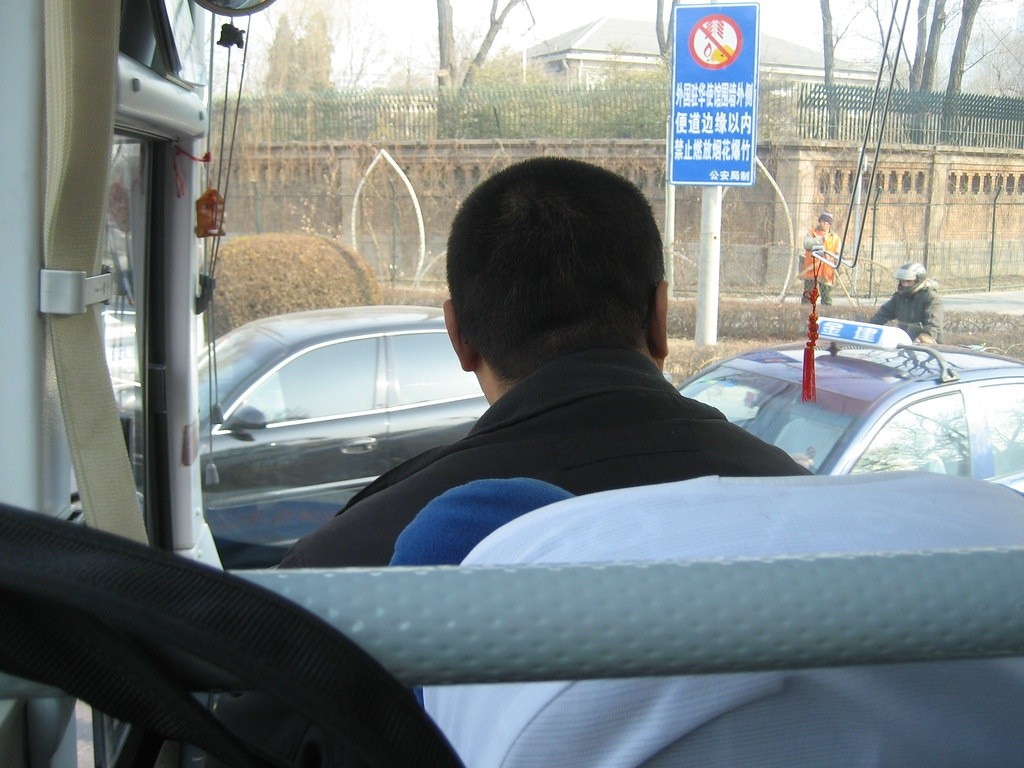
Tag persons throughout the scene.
[800,212,842,305]
[280,158,815,566]
[866,262,945,344]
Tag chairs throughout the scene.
[415,476,1024,768]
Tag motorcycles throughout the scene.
[904,320,986,352]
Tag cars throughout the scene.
[671,315,1024,495]
[112,302,491,549]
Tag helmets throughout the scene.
[896,263,928,298]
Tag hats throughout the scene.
[819,211,833,226]
